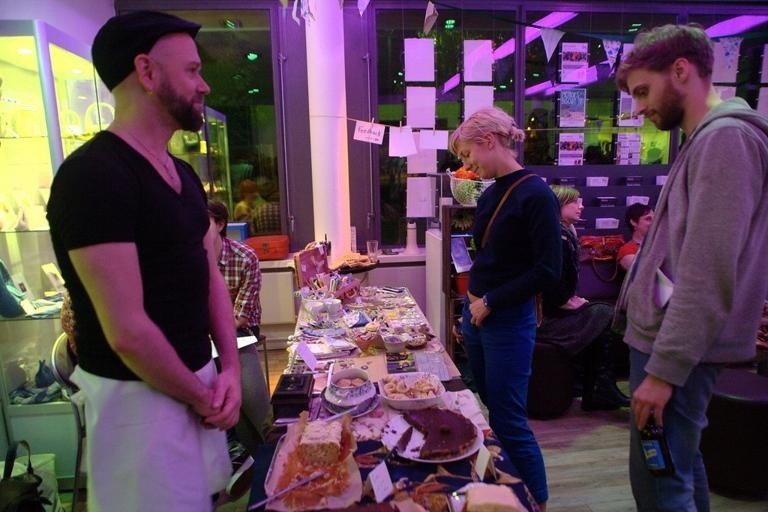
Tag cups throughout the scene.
[304,298,342,319]
[367,240,378,263]
[382,334,408,355]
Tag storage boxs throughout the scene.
[244,236,290,260]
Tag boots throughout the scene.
[596,374,631,407]
[580,383,621,411]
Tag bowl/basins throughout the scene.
[449,178,498,208]
[377,371,445,410]
[326,367,375,411]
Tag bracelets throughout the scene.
[481,292,493,312]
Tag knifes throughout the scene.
[385,426,413,461]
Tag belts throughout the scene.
[466,289,482,304]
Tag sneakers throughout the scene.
[225,443,256,501]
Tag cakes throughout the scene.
[467,483,525,512]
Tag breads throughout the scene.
[403,408,477,459]
[300,420,343,466]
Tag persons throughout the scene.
[617,203,657,273]
[43,10,245,512]
[232,177,260,236]
[205,199,281,499]
[252,181,281,236]
[449,107,565,511]
[537,183,635,411]
[612,24,768,512]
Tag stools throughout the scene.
[528,342,575,418]
[255,333,271,398]
[703,370,768,500]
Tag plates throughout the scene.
[381,412,482,464]
[319,387,379,419]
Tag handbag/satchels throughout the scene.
[579,233,625,283]
[0,440,47,512]
[534,286,544,328]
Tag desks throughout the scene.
[258,251,298,325]
[248,278,540,511]
[334,257,379,288]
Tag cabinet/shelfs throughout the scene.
[442,203,478,359]
[1,25,231,487]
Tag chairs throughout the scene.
[51,330,90,510]
